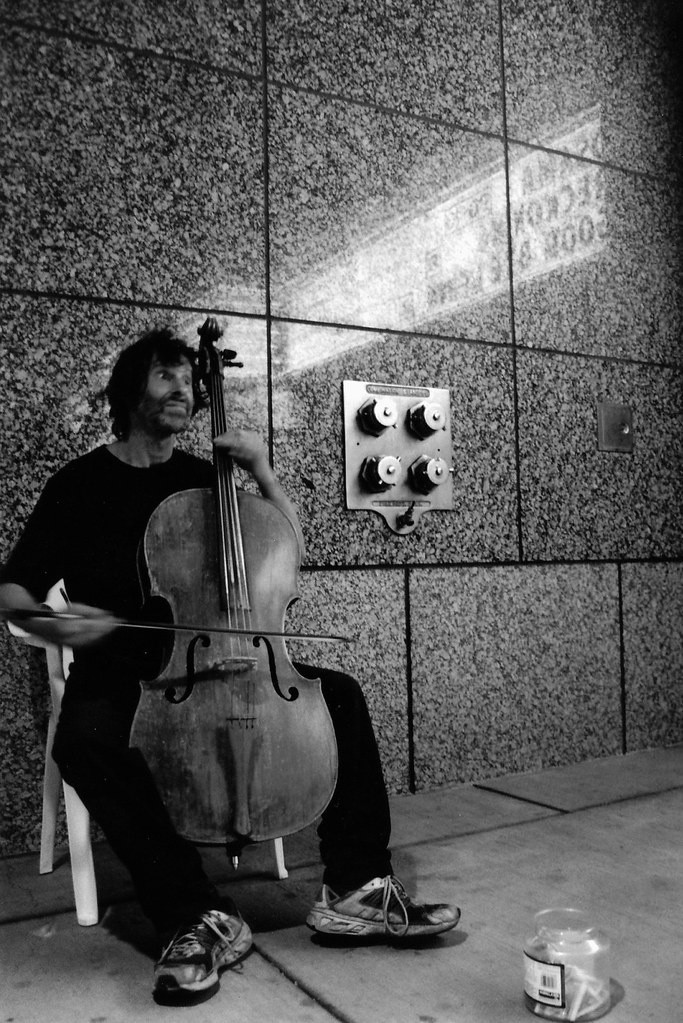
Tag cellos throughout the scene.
[127,316,341,873]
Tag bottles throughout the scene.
[524,906,612,1023]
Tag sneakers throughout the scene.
[304,873,461,937]
[151,896,251,993]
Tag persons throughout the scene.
[0,330,462,993]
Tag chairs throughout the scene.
[8,581,289,926]
